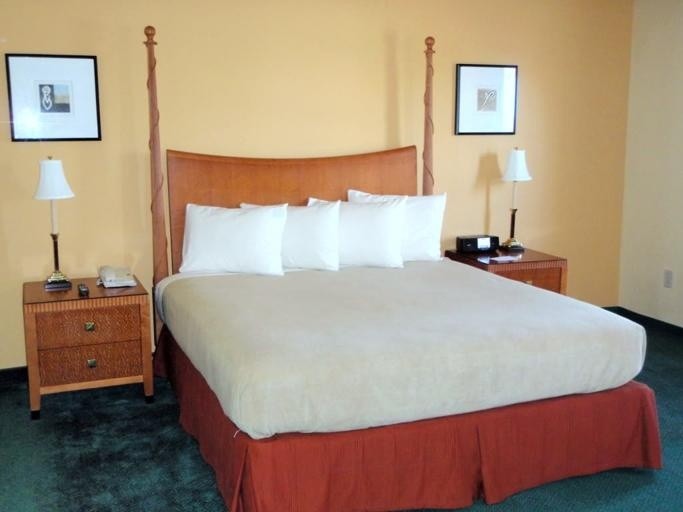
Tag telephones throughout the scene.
[97,265,137,288]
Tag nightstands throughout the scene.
[445,248,569,298]
[24,274,154,420]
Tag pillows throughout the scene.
[179,201,286,276]
[240,202,338,272]
[348,188,449,261]
[306,195,408,267]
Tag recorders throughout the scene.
[457,235,499,252]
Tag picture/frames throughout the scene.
[453,63,517,135]
[6,52,101,140]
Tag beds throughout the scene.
[145,24,663,512]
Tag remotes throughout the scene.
[78,284,88,295]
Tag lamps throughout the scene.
[502,149,533,251]
[31,154,75,292]
